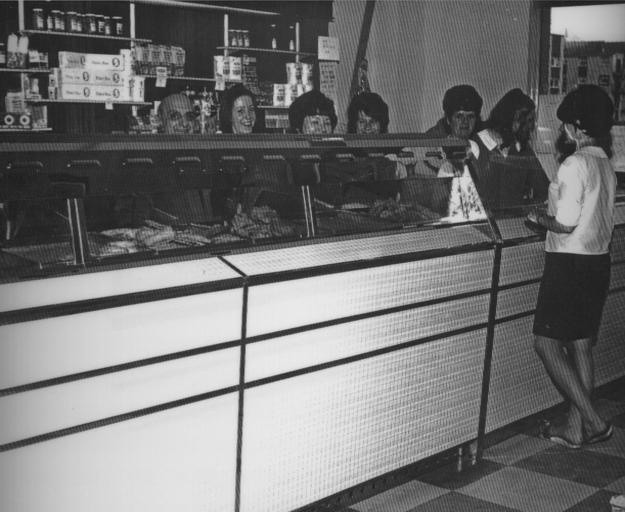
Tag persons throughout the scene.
[347,90,392,135]
[213,82,264,134]
[524,77,621,451]
[284,92,341,135]
[426,82,488,139]
[462,83,541,210]
[154,93,202,136]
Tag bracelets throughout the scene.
[537,214,547,224]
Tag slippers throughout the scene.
[535,418,581,450]
[582,424,612,443]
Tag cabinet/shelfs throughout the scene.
[1,127,499,512]
[0,0,317,132]
